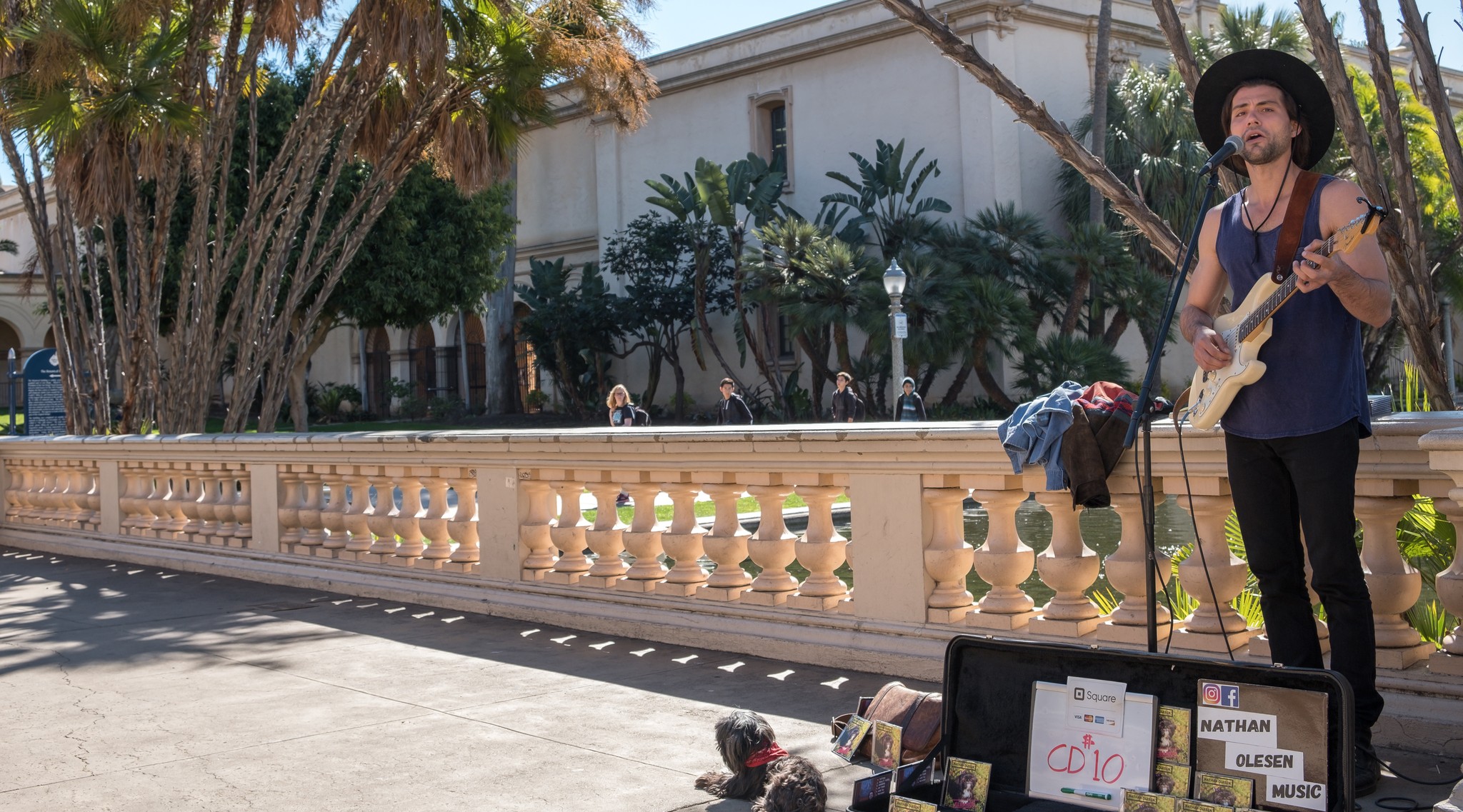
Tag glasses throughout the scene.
[614,393,624,396]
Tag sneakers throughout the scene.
[1354,729,1381,799]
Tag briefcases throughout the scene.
[844,632,1355,812]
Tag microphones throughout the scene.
[1198,135,1244,176]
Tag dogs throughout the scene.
[694,707,828,812]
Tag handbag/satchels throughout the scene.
[830,678,943,765]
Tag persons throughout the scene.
[607,385,636,426]
[831,372,857,423]
[1178,49,1392,800]
[716,378,753,425]
[894,377,927,422]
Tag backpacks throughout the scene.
[620,403,651,426]
[843,391,865,422]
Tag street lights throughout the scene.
[882,256,907,420]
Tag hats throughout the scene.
[1193,49,1336,176]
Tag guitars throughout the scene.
[1189,186,1391,431]
